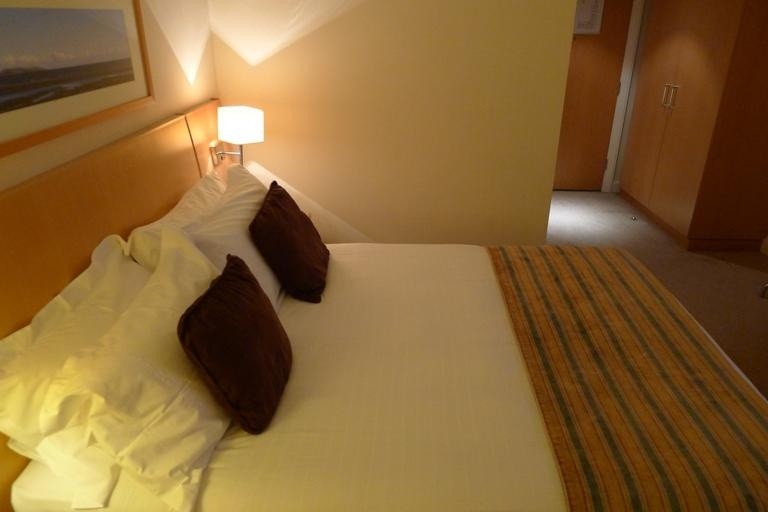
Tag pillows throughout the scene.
[180,164,288,315]
[248,180,332,304]
[176,253,294,434]
[3,234,153,460]
[123,168,227,273]
[37,225,235,512]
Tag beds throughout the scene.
[2,97,765,512]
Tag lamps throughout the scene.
[216,104,266,146]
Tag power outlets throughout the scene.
[208,140,226,166]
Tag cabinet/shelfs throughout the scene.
[617,0,768,254]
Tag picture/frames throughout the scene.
[0,2,157,160]
[573,0,605,35]
